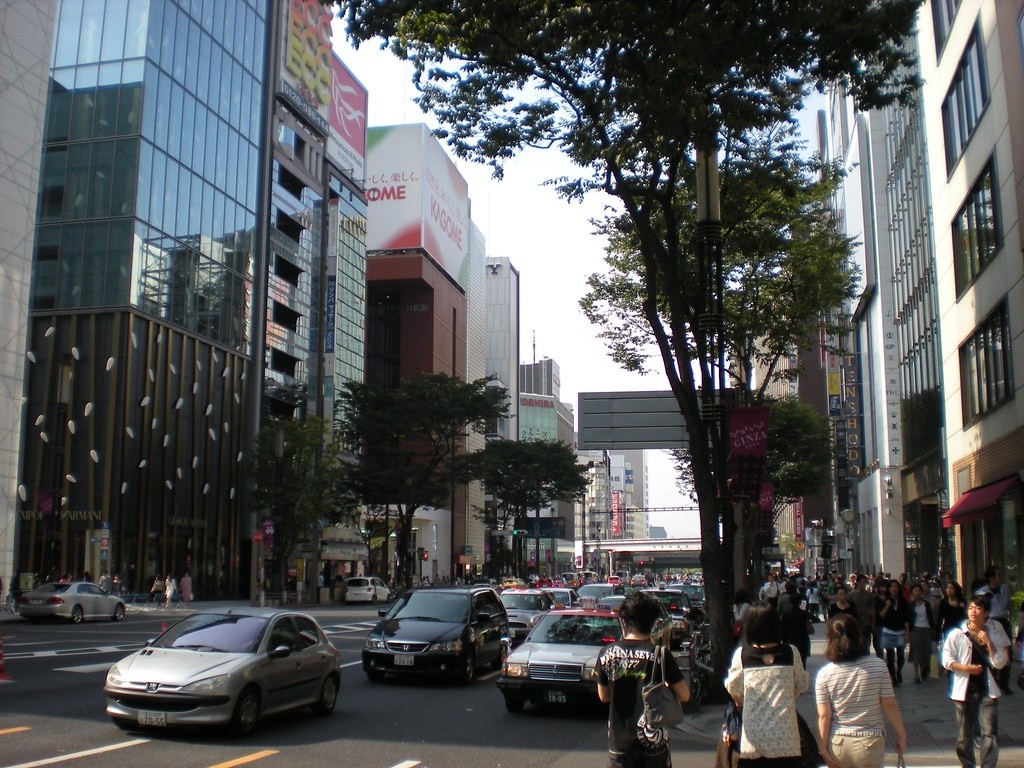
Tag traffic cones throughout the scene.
[0,634,9,680]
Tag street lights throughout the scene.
[608,550,612,577]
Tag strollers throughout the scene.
[716,703,822,768]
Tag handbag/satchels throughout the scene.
[807,619,815,635]
[930,653,944,680]
[641,646,685,728]
[733,620,741,638]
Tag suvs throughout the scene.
[361,583,512,688]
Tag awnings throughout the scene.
[941,474,1020,526]
[296,552,368,561]
[789,560,804,567]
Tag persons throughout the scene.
[941,594,1011,768]
[759,569,968,687]
[388,574,397,589]
[540,572,560,580]
[9,569,21,615]
[179,572,192,607]
[165,573,177,609]
[972,565,1024,695]
[650,579,655,587]
[724,602,809,768]
[814,614,907,768]
[0,577,3,611]
[421,575,465,586]
[592,592,690,768]
[99,570,125,597]
[33,571,39,588]
[317,572,324,603]
[151,574,164,609]
[666,572,678,584]
[46,565,89,583]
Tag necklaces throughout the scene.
[968,625,985,643]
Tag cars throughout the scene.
[576,583,627,609]
[600,595,674,652]
[499,589,565,642]
[502,579,523,590]
[496,608,630,713]
[19,582,126,625]
[608,571,648,589]
[345,576,391,604]
[669,582,707,616]
[103,607,341,738]
[542,587,583,611]
[537,572,600,589]
[642,589,696,644]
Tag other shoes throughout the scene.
[892,677,899,687]
[896,671,902,684]
[914,677,921,684]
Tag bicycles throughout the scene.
[690,644,714,699]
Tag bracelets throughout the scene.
[819,751,828,755]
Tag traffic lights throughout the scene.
[576,556,581,566]
[513,531,525,536]
